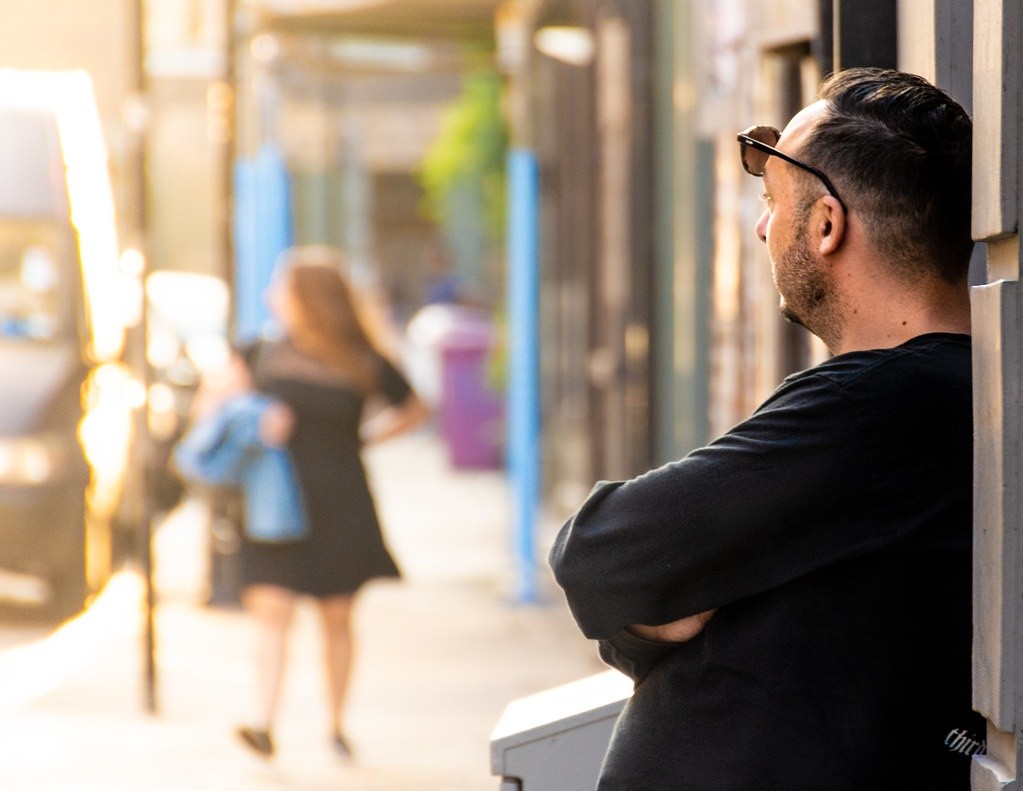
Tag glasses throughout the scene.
[736,124,847,217]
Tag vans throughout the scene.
[0,67,138,611]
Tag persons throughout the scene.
[549,68,988,791]
[215,249,426,760]
